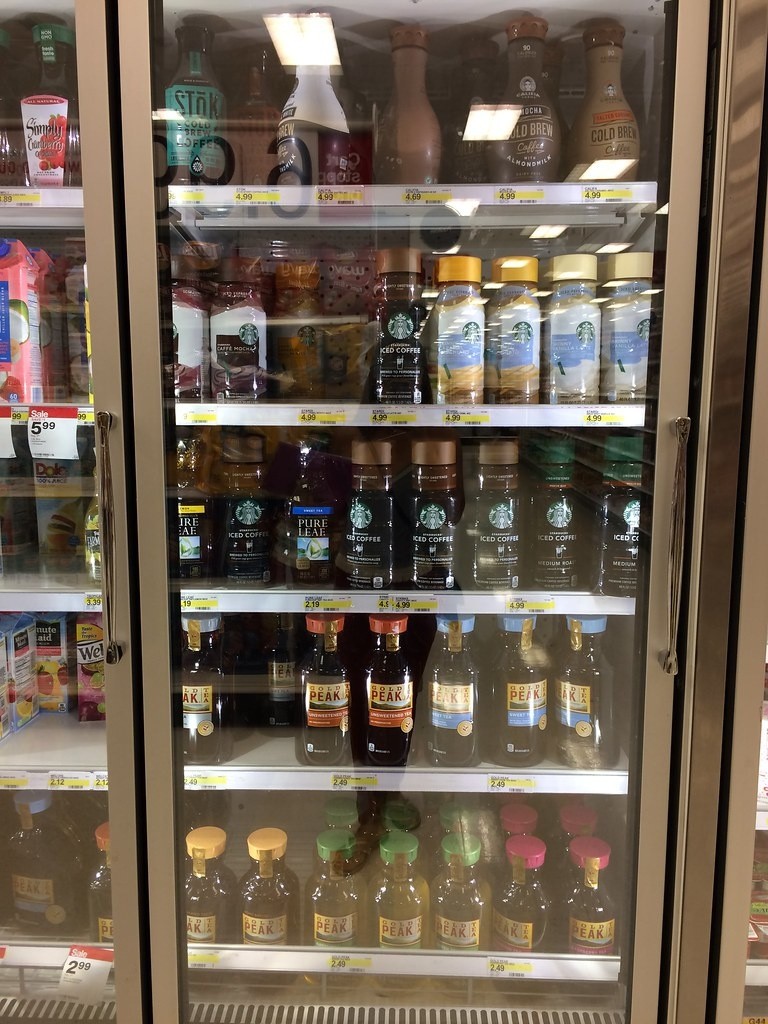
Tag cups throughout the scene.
[20,97,68,187]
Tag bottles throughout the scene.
[307,800,616,1002]
[234,26,371,188]
[178,826,238,1000]
[166,26,226,188]
[164,240,659,602]
[234,828,299,994]
[0,790,115,947]
[374,24,444,187]
[172,613,618,776]
[564,17,641,183]
[450,16,585,183]
